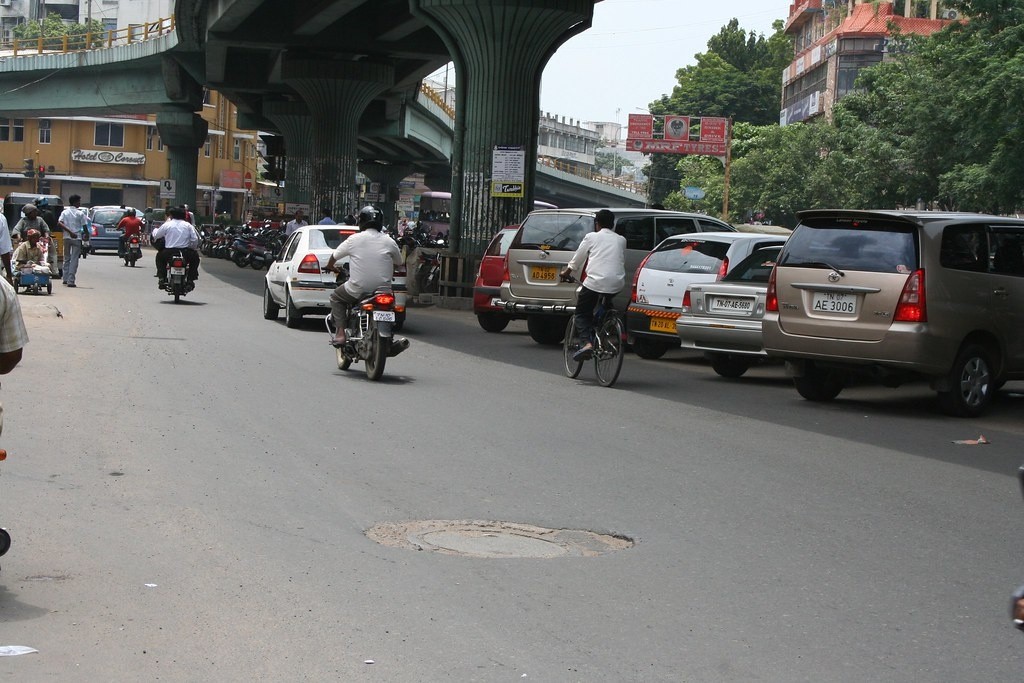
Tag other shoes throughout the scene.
[160,279,165,290]
[68,284,76,287]
[188,280,194,287]
[63,281,67,284]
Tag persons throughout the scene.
[560,209,627,360]
[152,207,200,290]
[10,204,50,291]
[0,212,13,286]
[179,203,195,225]
[16,229,44,271]
[116,209,143,258]
[140,207,153,224]
[324,206,403,344]
[0,275,30,375]
[318,209,336,225]
[286,210,308,238]
[57,194,89,287]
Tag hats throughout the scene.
[27,229,41,237]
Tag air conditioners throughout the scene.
[38,120,50,129]
[942,9,958,20]
[0,0,12,7]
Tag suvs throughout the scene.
[494,207,741,347]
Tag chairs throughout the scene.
[993,239,1024,274]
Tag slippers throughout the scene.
[573,349,593,361]
[329,337,344,345]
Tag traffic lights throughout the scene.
[38,164,45,178]
[21,159,34,178]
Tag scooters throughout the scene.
[379,222,450,293]
[199,218,290,271]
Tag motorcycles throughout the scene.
[163,249,193,301]
[0,192,65,268]
[319,263,410,381]
[116,228,145,267]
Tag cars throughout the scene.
[762,205,1024,417]
[472,223,523,333]
[624,232,791,348]
[78,205,169,254]
[677,244,785,354]
[261,225,386,329]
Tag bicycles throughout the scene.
[555,272,629,390]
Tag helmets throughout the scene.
[343,215,355,224]
[146,207,153,213]
[359,206,383,231]
[127,209,136,215]
[184,204,188,210]
